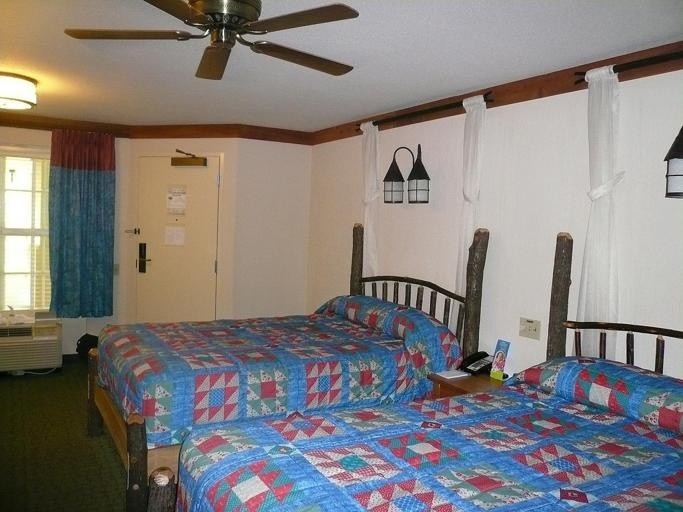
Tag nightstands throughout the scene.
[426,366,506,399]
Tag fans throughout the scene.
[62,0,359,82]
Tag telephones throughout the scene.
[457,351,495,376]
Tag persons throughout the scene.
[491,351,505,369]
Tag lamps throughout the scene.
[0,70,38,114]
[382,143,430,205]
[663,126,682,197]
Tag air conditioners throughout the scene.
[0,321,63,376]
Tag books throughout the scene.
[434,369,470,379]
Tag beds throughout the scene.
[145,231,682,510]
[87,222,489,510]
